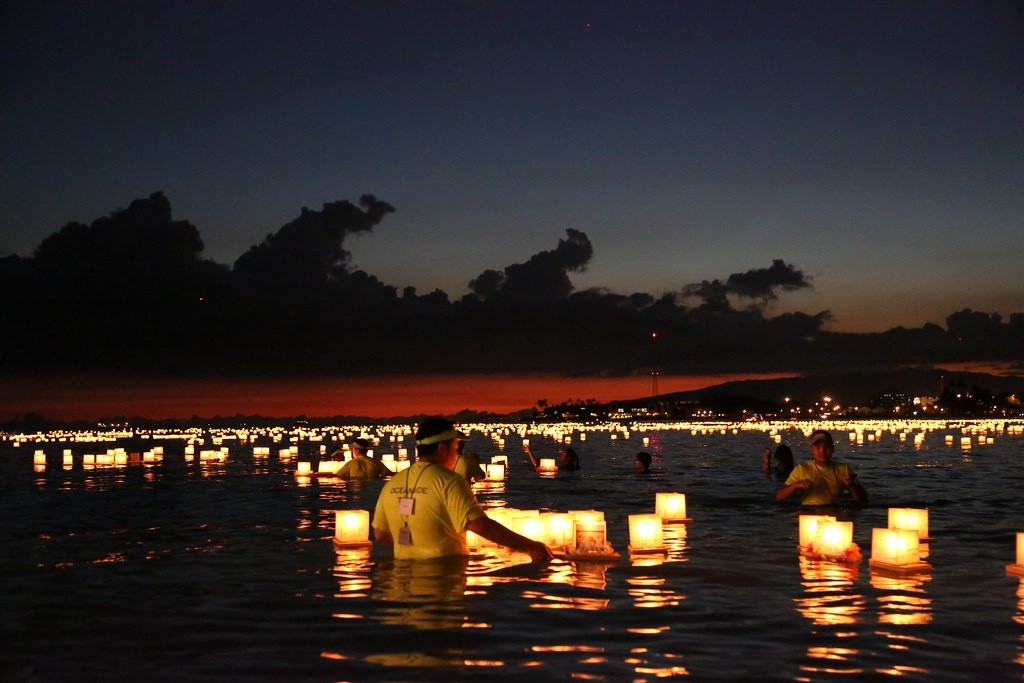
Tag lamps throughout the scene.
[0,410,1024,578]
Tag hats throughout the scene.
[416,427,473,445]
[809,430,833,446]
[349,442,369,451]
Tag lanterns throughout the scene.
[0,419,1024,590]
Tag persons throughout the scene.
[634,452,652,472]
[524,445,581,472]
[764,444,794,475]
[335,438,398,478]
[776,430,868,505]
[371,417,555,562]
[450,430,486,485]
